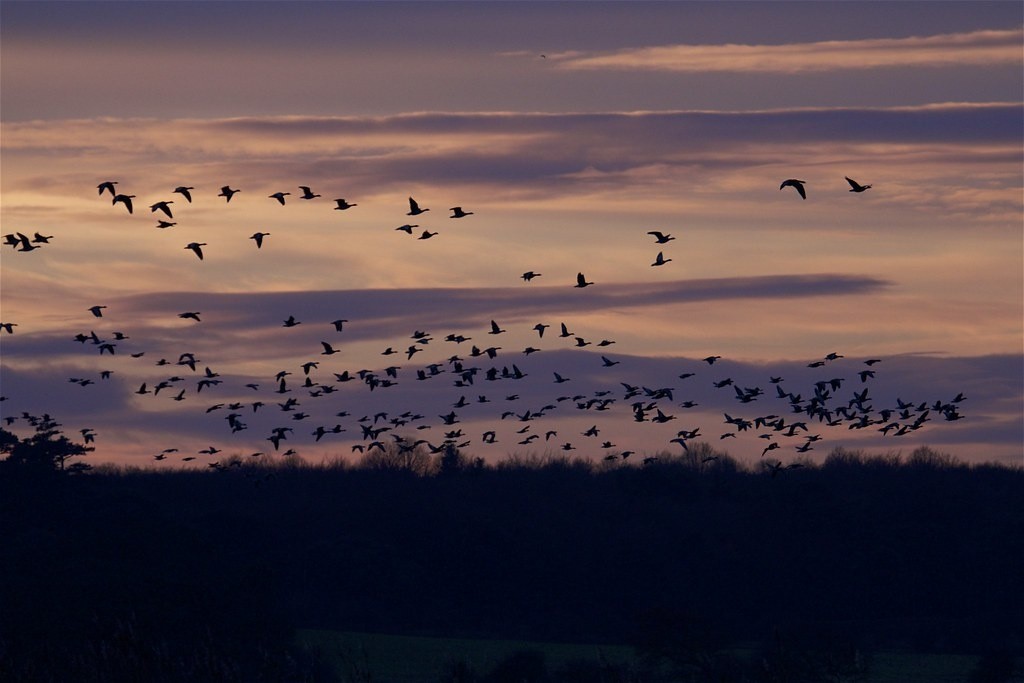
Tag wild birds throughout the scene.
[519,271,542,282]
[96,180,476,264]
[780,179,807,200]
[1,231,55,252]
[646,230,676,267]
[0,305,972,477]
[573,271,595,288]
[845,175,872,193]
